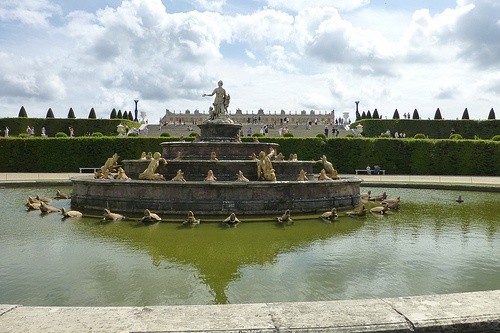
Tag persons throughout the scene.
[240,116,406,174]
[101,81,334,182]
[3,117,200,142]
[450,128,456,135]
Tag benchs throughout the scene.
[79,168,101,172]
[355,170,386,175]
